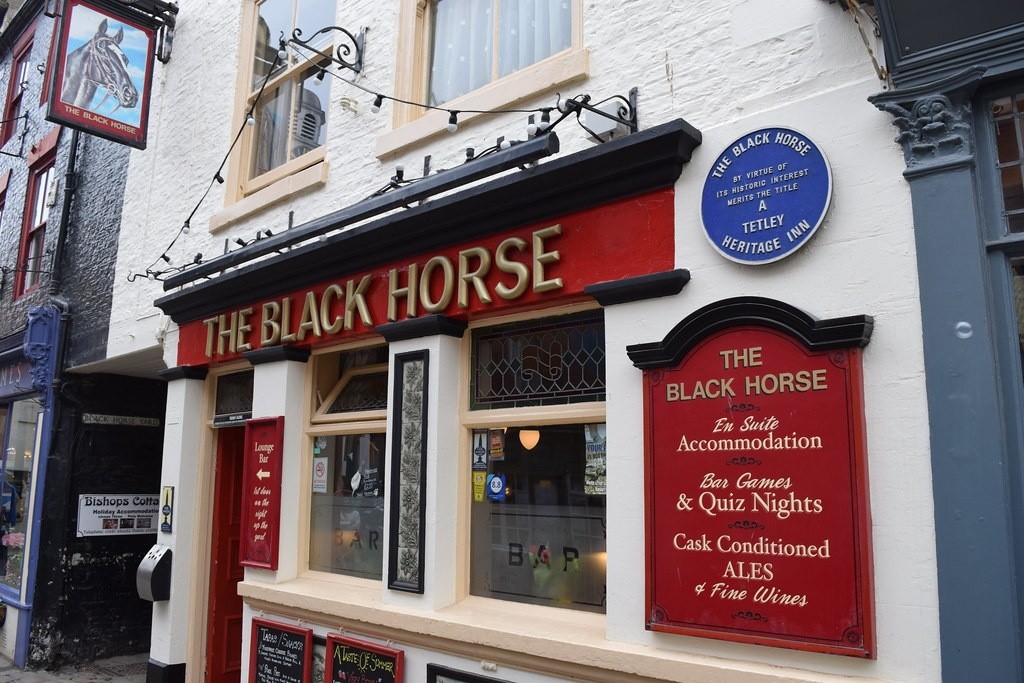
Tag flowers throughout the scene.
[3,530,25,549]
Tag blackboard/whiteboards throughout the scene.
[248,617,313,683]
[324,633,403,683]
